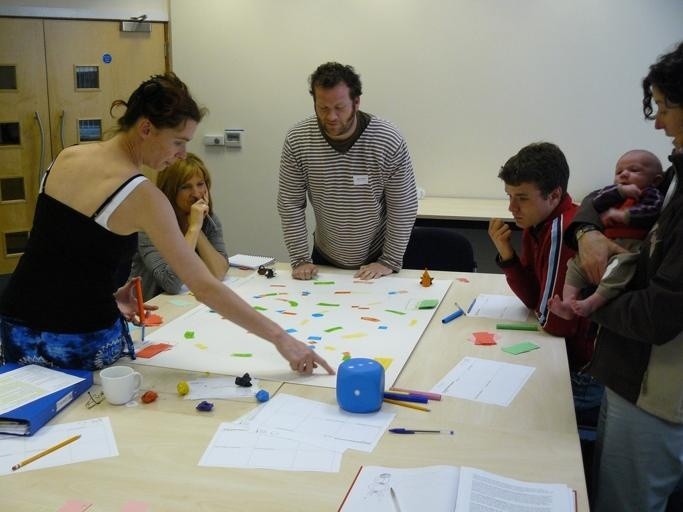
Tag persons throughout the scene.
[277,61,418,281]
[578,43,683,512]
[547,150,663,321]
[127,152,229,303]
[0,71,335,376]
[487,142,604,429]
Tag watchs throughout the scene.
[575,225,597,240]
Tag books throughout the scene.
[338,465,578,512]
[228,254,275,270]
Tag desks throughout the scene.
[0,261,590,512]
[402,197,525,274]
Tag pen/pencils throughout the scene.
[134,277,144,325]
[442,309,463,324]
[390,487,401,512]
[467,298,476,313]
[383,387,441,412]
[388,428,454,435]
[496,323,538,331]
[12,434,82,471]
[455,302,466,315]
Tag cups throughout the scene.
[100,366,142,405]
[415,186,425,199]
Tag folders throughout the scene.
[0,362,94,436]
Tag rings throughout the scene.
[202,205,206,207]
